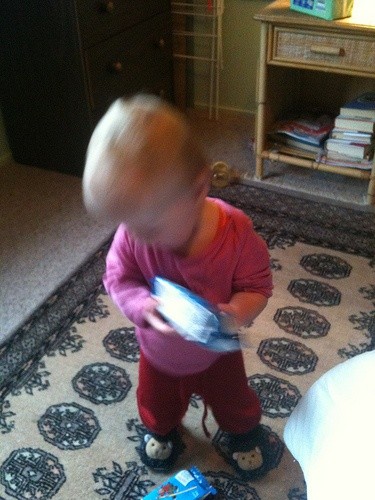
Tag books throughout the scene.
[275,90,375,158]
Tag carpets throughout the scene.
[0,192,375,500]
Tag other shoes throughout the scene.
[225,424,269,481]
[134,426,185,473]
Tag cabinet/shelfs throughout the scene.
[253,0,375,205]
[0,0,173,178]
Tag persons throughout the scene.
[82,94,274,481]
